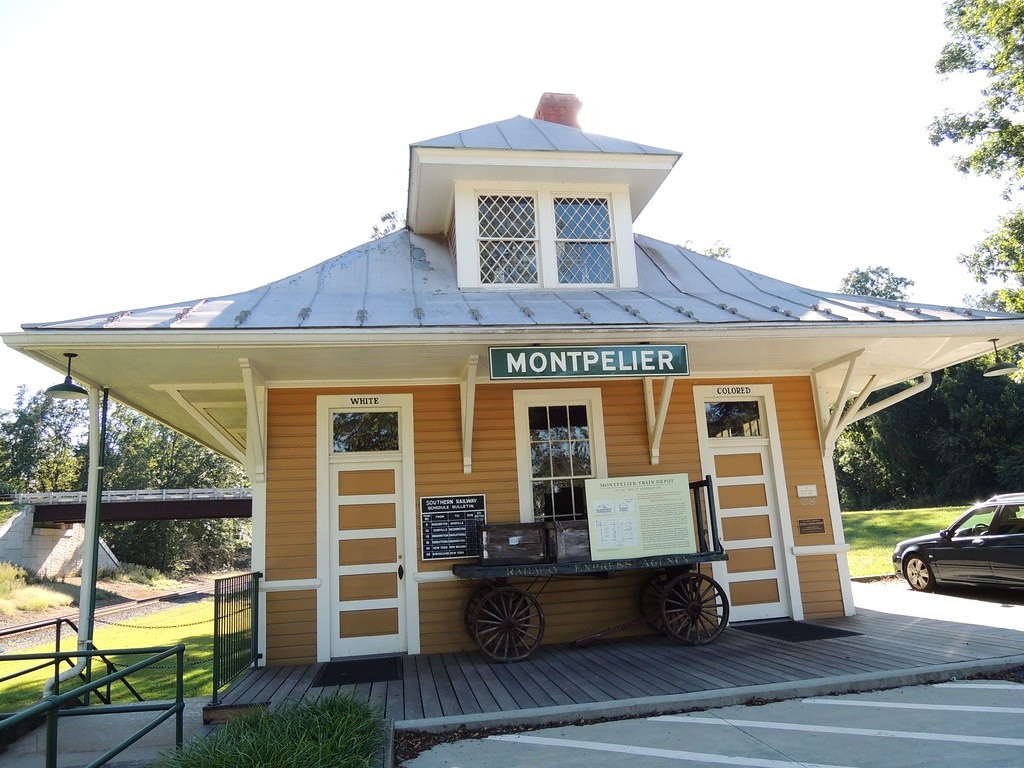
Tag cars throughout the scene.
[892,493,1024,593]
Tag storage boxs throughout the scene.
[545,520,591,564]
[476,522,544,567]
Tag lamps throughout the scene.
[43,352,90,399]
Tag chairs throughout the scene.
[1004,505,1024,534]
